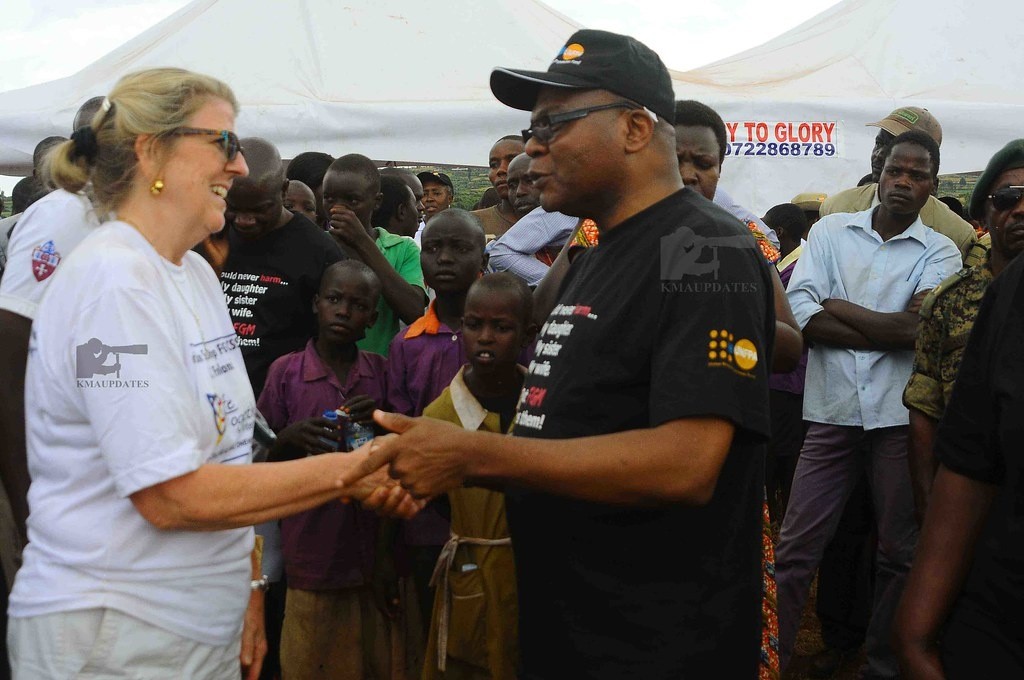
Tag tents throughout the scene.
[668,0,1022,217]
[0,0,586,176]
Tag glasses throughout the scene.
[520,98,659,145]
[159,127,245,162]
[986,186,1024,210]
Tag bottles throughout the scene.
[344,409,373,453]
[308,410,339,457]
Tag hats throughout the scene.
[792,195,827,211]
[864,105,942,149]
[967,140,1024,221]
[416,172,452,187]
[490,29,676,125]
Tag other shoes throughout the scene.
[811,646,849,678]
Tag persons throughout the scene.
[334,30,773,680]
[6,67,401,680]
[0,96,118,548]
[213,135,562,680]
[819,107,977,261]
[489,99,824,373]
[890,250,1024,680]
[901,140,1024,528]
[775,130,963,674]
